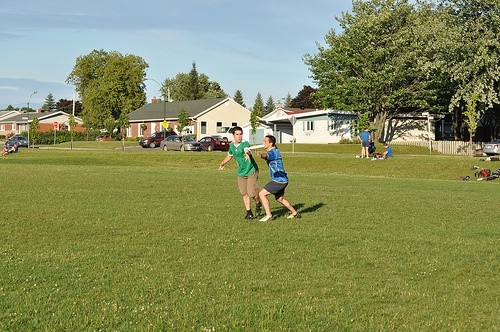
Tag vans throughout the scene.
[217,125,238,141]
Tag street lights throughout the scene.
[143,78,167,153]
[26,90,40,149]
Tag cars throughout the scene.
[198,136,230,152]
[482,140,500,155]
[5,137,29,147]
[161,135,201,152]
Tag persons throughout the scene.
[357,128,371,159]
[256,135,301,221]
[372,143,395,160]
[1,142,18,155]
[219,126,263,219]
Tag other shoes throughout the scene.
[255,202,262,218]
[246,213,253,219]
[259,215,273,222]
[287,213,300,219]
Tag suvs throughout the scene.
[139,131,176,148]
